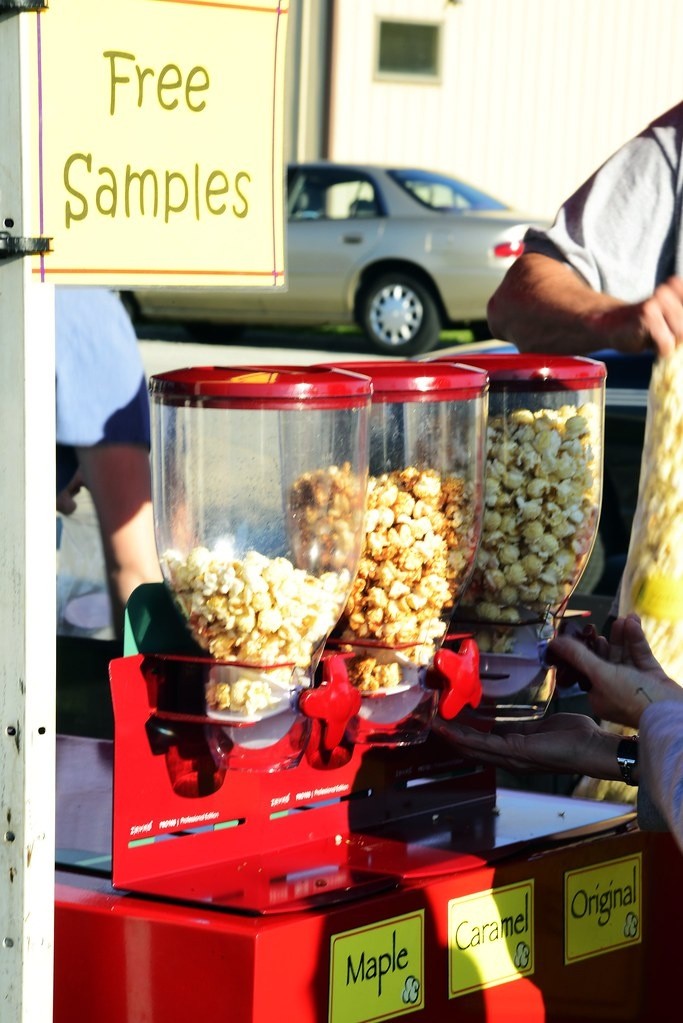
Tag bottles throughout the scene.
[311,360,490,692]
[433,350,605,659]
[147,366,375,727]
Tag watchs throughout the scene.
[616,735,639,786]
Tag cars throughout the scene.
[327,335,659,600]
[116,159,561,358]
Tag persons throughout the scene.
[54,283,164,606]
[427,614,683,852]
[485,101,683,357]
